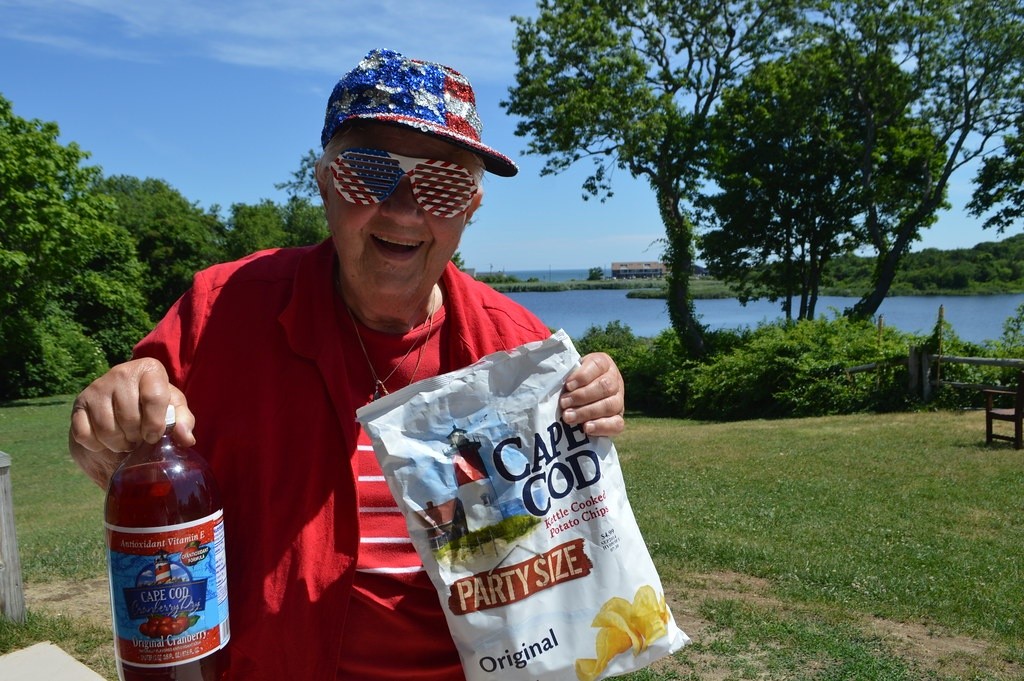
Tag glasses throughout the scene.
[326,147,477,220]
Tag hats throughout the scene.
[321,48,521,178]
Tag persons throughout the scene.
[68,48,626,681]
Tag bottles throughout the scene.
[104,405,230,681]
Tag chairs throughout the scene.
[981,370,1024,450]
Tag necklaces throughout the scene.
[337,284,436,400]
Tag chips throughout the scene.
[574,586,671,681]
